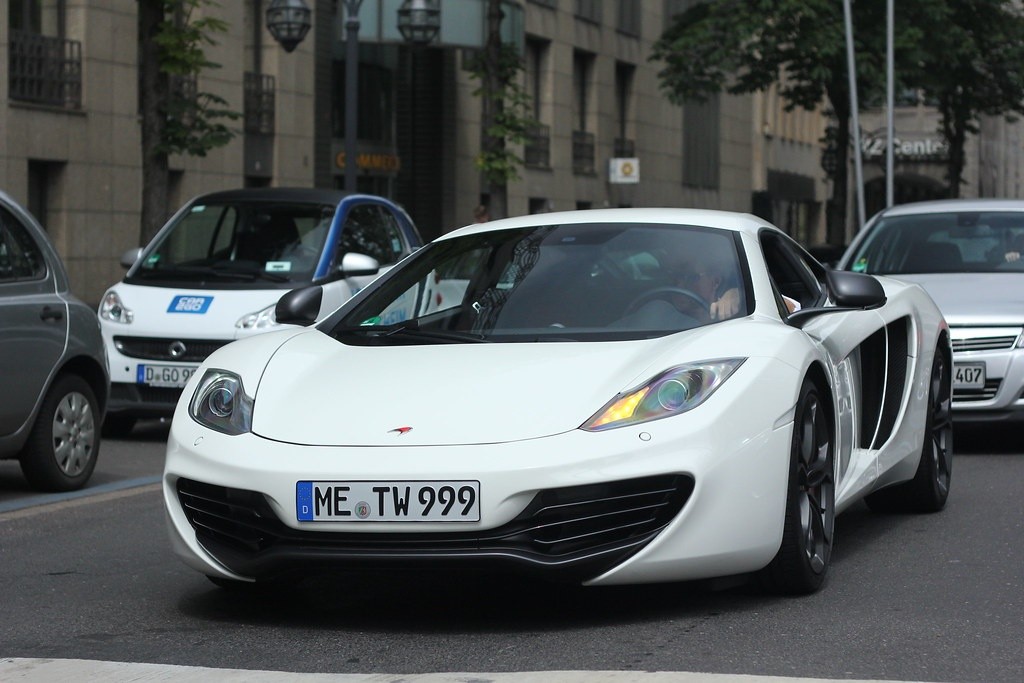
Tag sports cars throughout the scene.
[162,207,954,594]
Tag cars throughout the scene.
[835,198,1023,426]
[1,191,111,491]
[103,188,443,440]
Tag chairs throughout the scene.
[477,292,542,330]
[902,240,962,271]
[564,274,637,326]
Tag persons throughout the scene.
[676,249,802,324]
[1003,233,1024,262]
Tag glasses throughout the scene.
[675,271,713,285]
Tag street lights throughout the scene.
[267,0,441,193]
